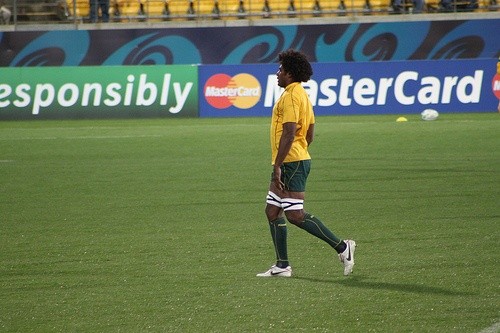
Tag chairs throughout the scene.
[1,0,499,25]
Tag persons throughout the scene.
[88,0,110,23]
[0,0,11,25]
[391,0,478,14]
[256,48,355,278]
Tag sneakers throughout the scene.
[338,239,355,275]
[256,265,292,277]
